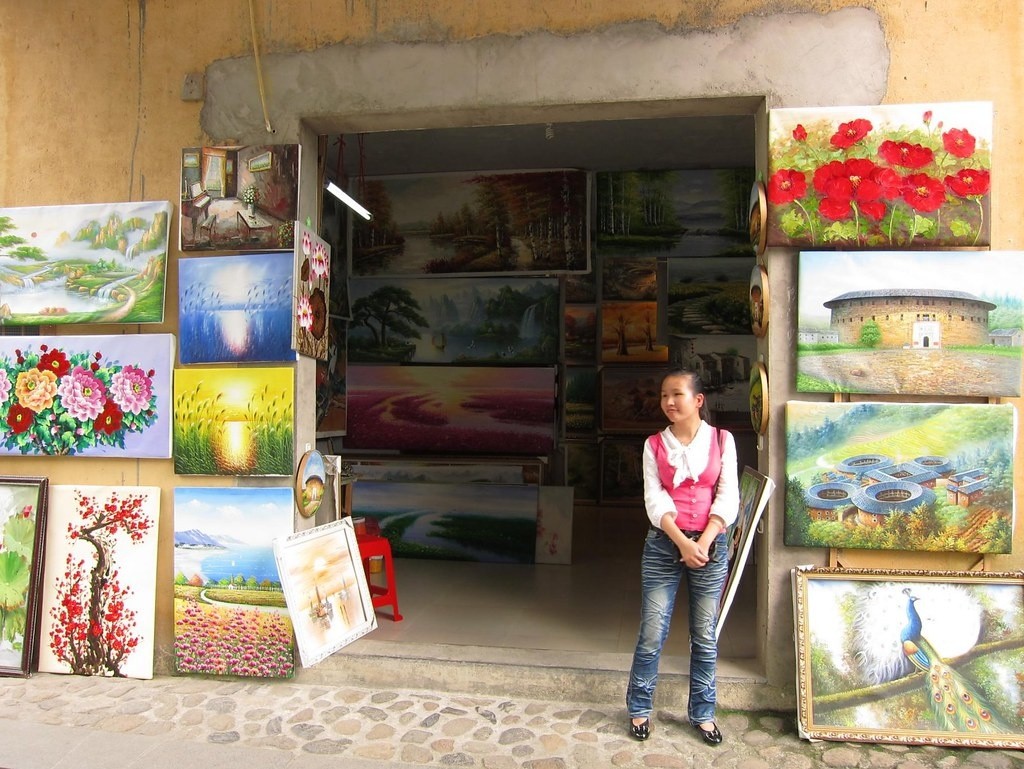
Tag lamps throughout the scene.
[323,177,375,223]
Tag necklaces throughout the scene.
[671,428,684,446]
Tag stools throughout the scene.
[356,531,403,621]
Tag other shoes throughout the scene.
[630,718,651,741]
[698,722,722,745]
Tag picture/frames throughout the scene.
[332,454,544,520]
[0,475,48,679]
[557,363,671,508]
[794,565,1024,751]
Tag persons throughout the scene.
[626,368,739,746]
